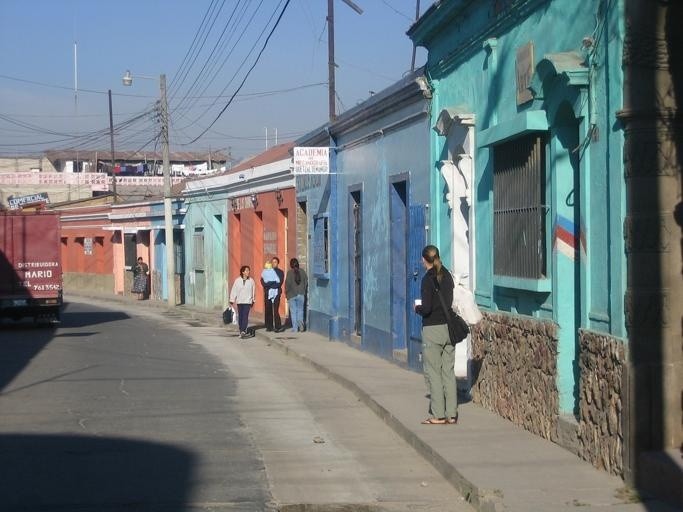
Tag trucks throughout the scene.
[1,210,65,327]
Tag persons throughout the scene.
[284,257,308,334]
[411,243,463,428]
[260,260,280,304]
[258,256,284,334]
[228,265,256,339]
[130,256,150,301]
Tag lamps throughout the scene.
[231,197,238,211]
[251,194,258,208]
[275,189,283,206]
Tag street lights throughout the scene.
[122,70,176,305]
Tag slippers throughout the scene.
[421,418,456,424]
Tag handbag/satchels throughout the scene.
[448,308,470,346]
